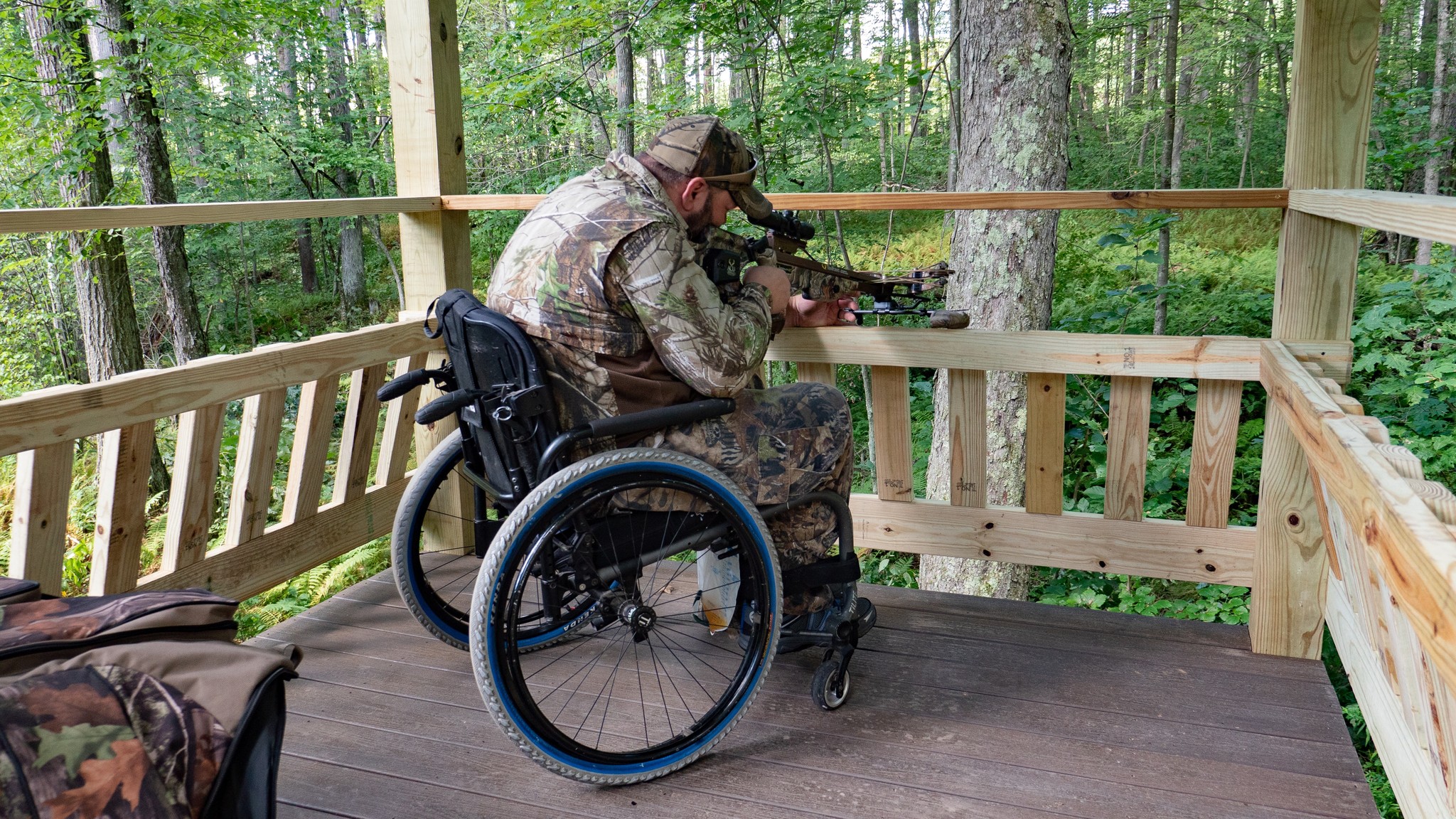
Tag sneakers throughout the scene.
[738,596,878,653]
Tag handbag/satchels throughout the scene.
[2,574,302,818]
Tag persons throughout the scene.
[486,113,878,657]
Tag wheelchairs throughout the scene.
[372,290,879,788]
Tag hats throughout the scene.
[646,114,774,219]
